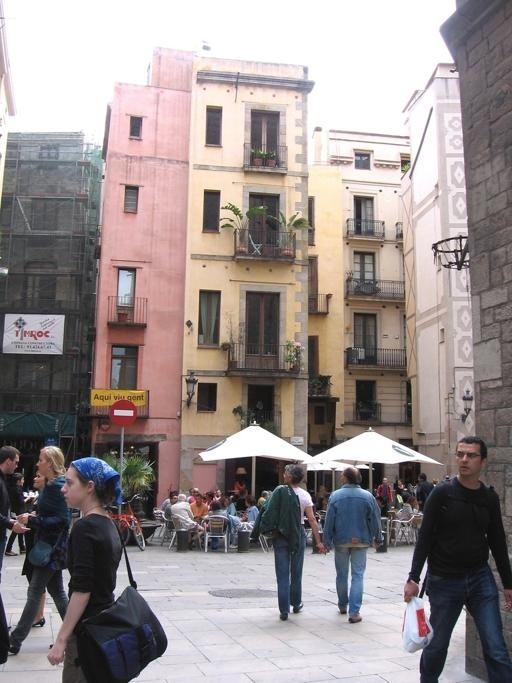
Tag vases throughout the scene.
[289,367,300,373]
[117,312,129,321]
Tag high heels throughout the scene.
[32,617,45,627]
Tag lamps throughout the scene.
[459,387,474,423]
[184,371,199,408]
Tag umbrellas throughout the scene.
[306,461,375,491]
[300,426,445,495]
[199,420,312,499]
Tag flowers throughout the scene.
[283,336,306,367]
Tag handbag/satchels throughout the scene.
[74,586,167,682]
[401,594,434,653]
[27,540,53,568]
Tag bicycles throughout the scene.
[106,494,147,550]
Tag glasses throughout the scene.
[455,452,481,458]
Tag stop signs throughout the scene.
[109,398,138,427]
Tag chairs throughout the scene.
[304,509,326,545]
[149,505,271,551]
[381,509,424,546]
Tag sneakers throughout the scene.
[5,551,18,555]
[340,606,347,614]
[293,602,303,613]
[280,614,288,620]
[7,643,19,655]
[349,613,362,622]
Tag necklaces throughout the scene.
[84,503,102,516]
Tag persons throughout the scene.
[47,456,126,683]
[160,474,450,551]
[320,466,385,624]
[403,435,512,683]
[0,445,69,664]
[250,464,325,621]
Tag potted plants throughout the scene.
[216,200,314,258]
[100,451,161,544]
[229,317,239,369]
[249,144,280,167]
